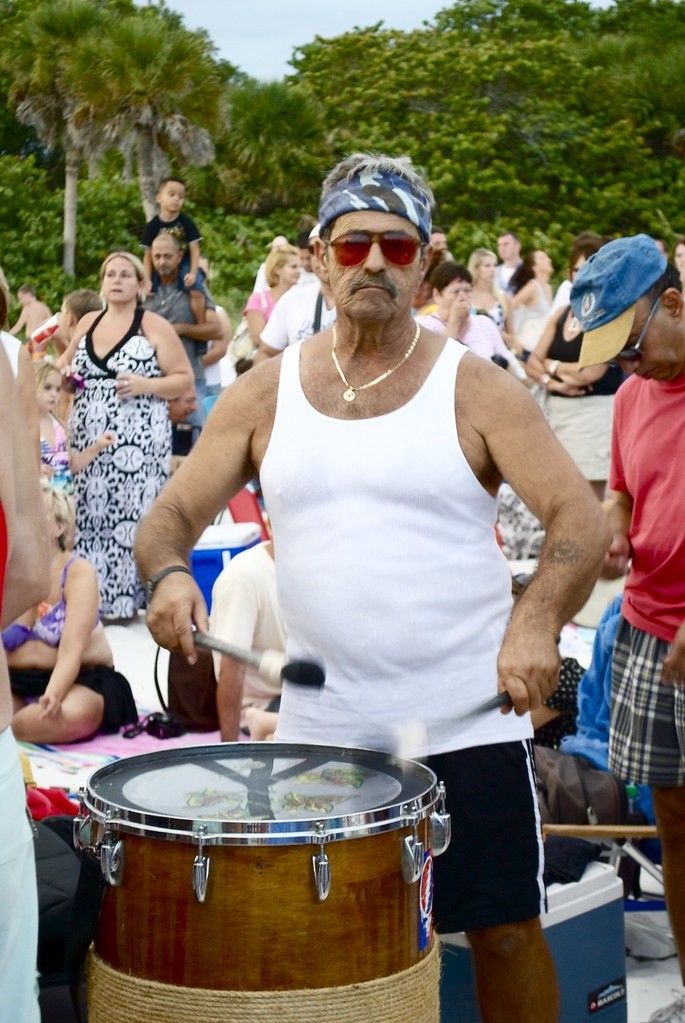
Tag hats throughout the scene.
[569,232,668,374]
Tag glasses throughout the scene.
[318,229,428,267]
[571,266,579,272]
[602,293,662,369]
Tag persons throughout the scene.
[0,179,333,744]
[0,269,53,1022]
[570,235,685,1023]
[233,220,685,498]
[141,149,613,1023]
[209,486,678,961]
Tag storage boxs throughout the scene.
[439,860,628,1023]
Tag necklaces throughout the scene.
[332,322,420,399]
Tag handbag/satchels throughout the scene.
[230,288,270,362]
[153,644,220,733]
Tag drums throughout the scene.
[73,742,450,1023]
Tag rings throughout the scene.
[125,381,129,386]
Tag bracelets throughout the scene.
[549,361,559,376]
[145,566,201,600]
[540,375,550,388]
